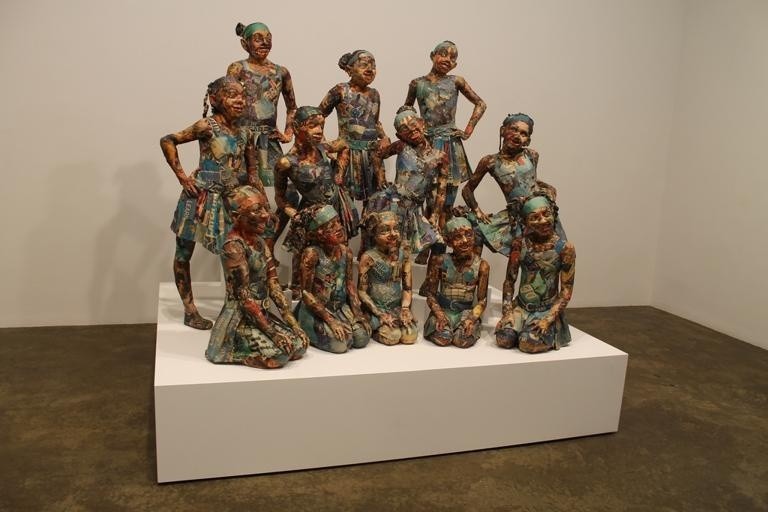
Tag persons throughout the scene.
[494,190,577,355]
[159,76,265,329]
[403,40,487,206]
[227,21,300,268]
[460,112,568,259]
[320,51,393,203]
[355,105,449,266]
[358,211,418,345]
[275,106,358,302]
[204,184,311,370]
[421,216,490,347]
[280,203,372,353]
